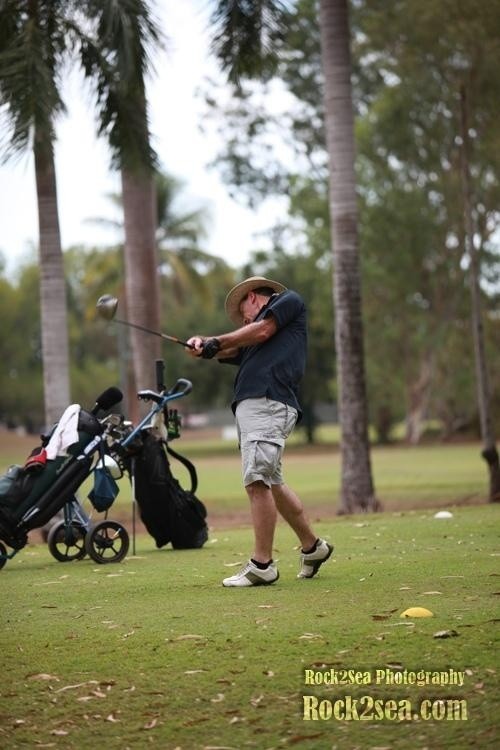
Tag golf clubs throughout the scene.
[97,414,134,448]
[97,294,195,349]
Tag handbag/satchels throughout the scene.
[88,467,119,512]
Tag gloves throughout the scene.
[197,338,220,360]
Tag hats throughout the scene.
[225,276,287,327]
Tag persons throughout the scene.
[184,276,333,588]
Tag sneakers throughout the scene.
[297,541,334,578]
[223,560,279,588]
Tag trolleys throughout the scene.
[1,379,193,567]
